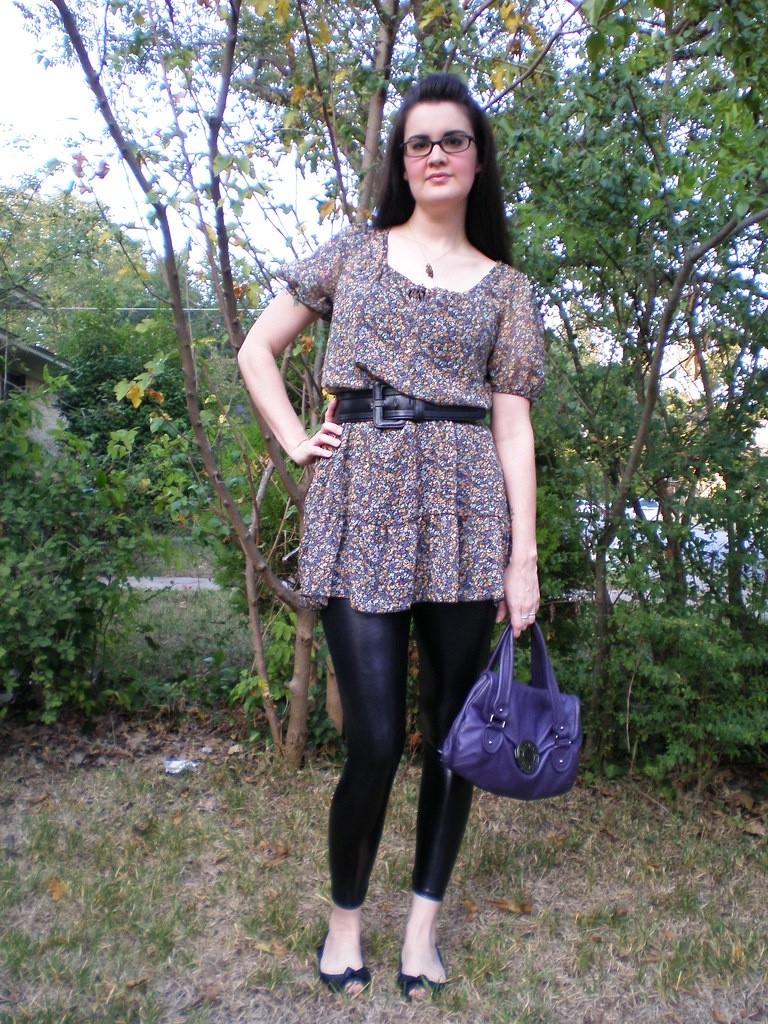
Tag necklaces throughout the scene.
[407,223,467,278]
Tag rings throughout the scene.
[522,617,528,619]
[529,613,535,615]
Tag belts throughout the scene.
[330,381,486,429]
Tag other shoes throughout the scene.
[398,943,447,1003]
[319,930,371,995]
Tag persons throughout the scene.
[238,76,540,1003]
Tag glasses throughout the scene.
[399,135,478,158]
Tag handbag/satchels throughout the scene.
[440,619,580,801]
